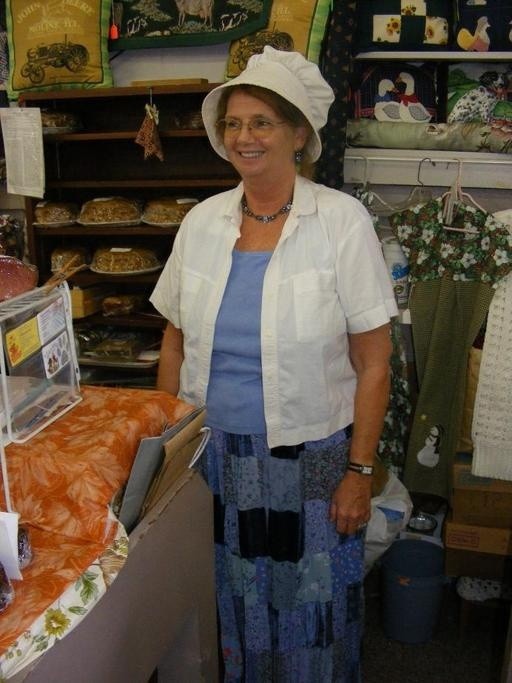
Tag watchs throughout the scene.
[348,460,376,476]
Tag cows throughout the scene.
[218,8,249,33]
[176,0,214,29]
[125,14,150,37]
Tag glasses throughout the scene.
[214,119,290,139]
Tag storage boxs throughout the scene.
[446,463,511,583]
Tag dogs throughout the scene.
[446,71,510,125]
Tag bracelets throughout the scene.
[240,195,295,227]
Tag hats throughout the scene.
[201,44,338,167]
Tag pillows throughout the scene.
[4,2,111,95]
[225,0,334,81]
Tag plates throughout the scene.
[30,217,182,279]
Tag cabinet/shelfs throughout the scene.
[17,82,242,374]
[334,47,512,186]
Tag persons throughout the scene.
[145,41,400,683]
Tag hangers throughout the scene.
[349,157,487,243]
